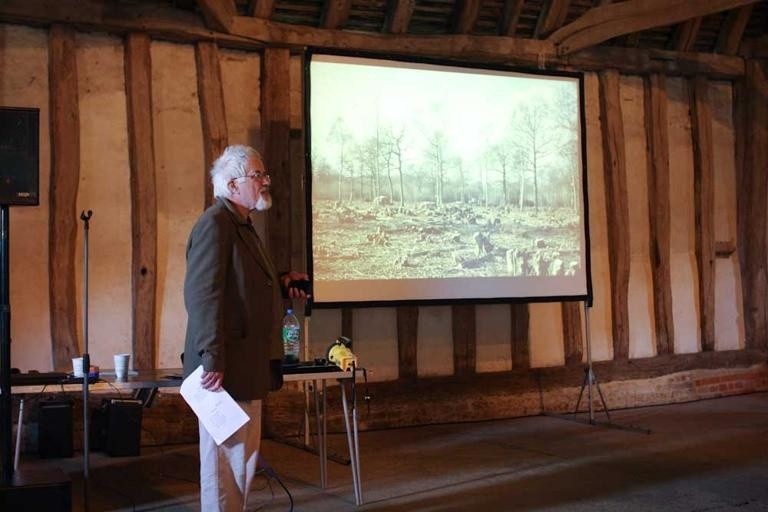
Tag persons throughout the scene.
[181,144,286,512]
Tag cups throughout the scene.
[113,353,131,380]
[71,356,85,377]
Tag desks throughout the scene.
[9,362,367,507]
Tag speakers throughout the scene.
[0,105,40,207]
[95,397,143,457]
[37,399,74,457]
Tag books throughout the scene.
[178,365,251,448]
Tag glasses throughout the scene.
[244,170,271,181]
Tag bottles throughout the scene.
[281,307,301,358]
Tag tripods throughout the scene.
[574,326,615,426]
